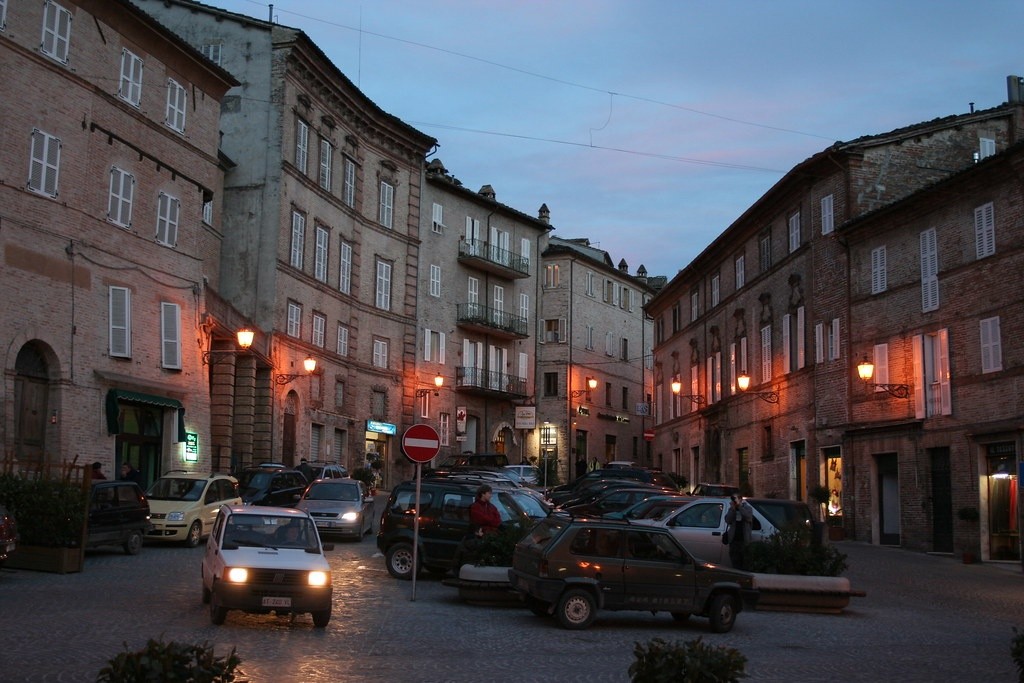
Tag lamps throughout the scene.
[737,370,780,404]
[672,377,705,404]
[275,353,317,385]
[203,327,256,367]
[857,355,908,399]
[415,372,444,398]
[570,376,598,398]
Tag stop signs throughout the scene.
[401,423,441,463]
[643,428,655,441]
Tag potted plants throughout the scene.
[438,516,539,608]
[0,462,93,574]
[807,483,844,542]
[744,520,866,613]
[349,453,385,497]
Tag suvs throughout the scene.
[140,469,239,549]
[293,460,351,479]
[227,463,308,508]
[507,508,760,635]
[47,479,151,555]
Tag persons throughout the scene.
[451,524,505,581]
[724,492,753,570]
[120,463,137,483]
[292,457,314,482]
[589,457,601,472]
[467,484,503,541]
[519,457,531,465]
[90,462,106,479]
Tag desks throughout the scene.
[993,533,1019,557]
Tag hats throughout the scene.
[300,458,307,461]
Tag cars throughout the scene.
[376,453,816,581]
[292,478,375,541]
[202,504,335,628]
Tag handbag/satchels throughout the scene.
[722,524,729,546]
